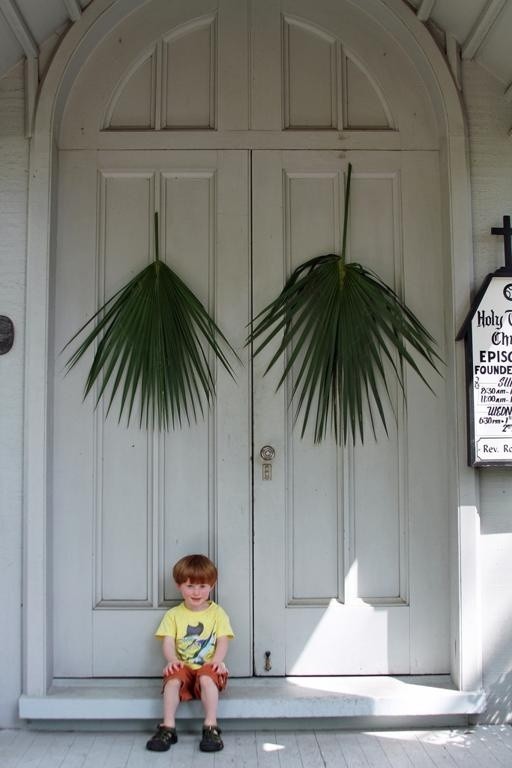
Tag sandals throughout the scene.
[198,724,225,752]
[146,723,179,753]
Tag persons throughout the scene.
[146,555,234,752]
